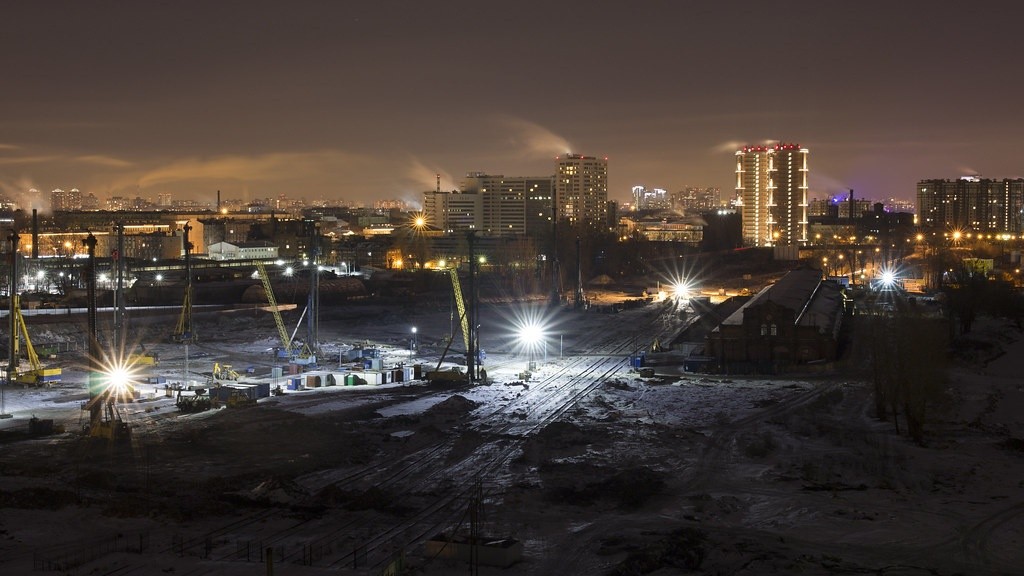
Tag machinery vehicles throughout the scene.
[212,363,246,381]
[5,232,62,388]
[449,261,486,365]
[167,221,199,344]
[77,232,131,444]
[252,259,317,370]
[550,206,591,312]
